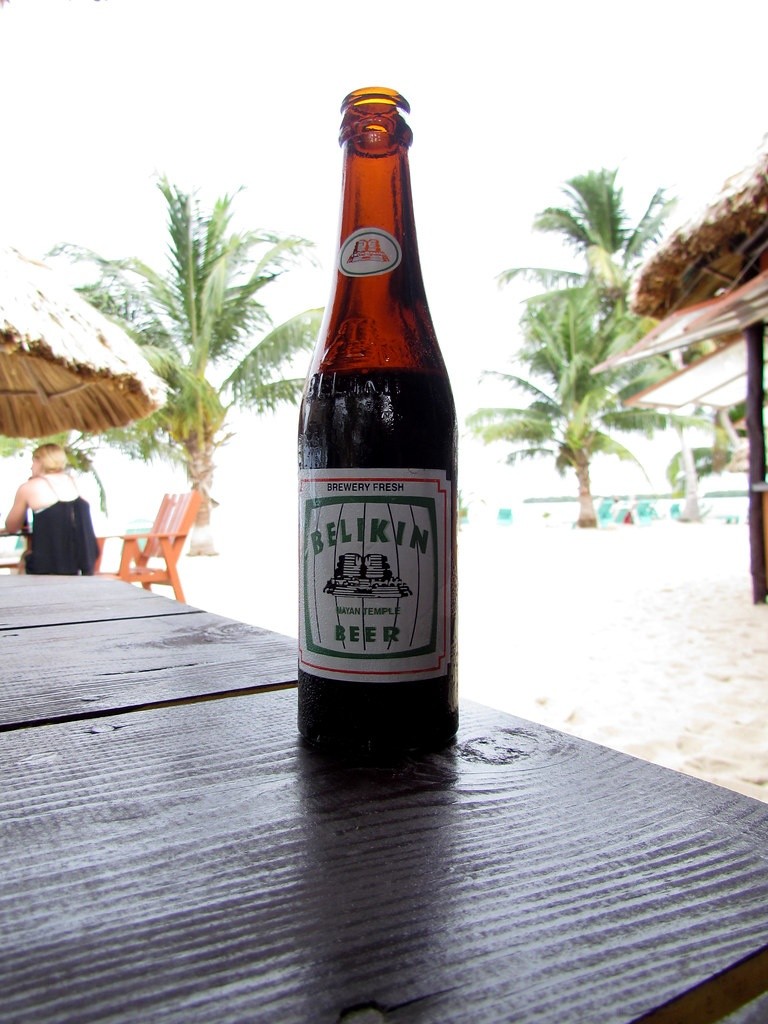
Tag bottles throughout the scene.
[290,84,460,754]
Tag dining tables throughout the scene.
[0,574,767,1024]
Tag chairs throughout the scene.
[96,489,202,607]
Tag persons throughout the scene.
[4,442,101,578]
